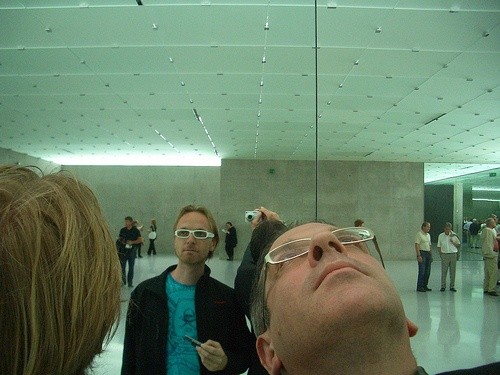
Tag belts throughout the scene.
[420,250,430,253]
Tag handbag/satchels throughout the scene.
[147,232,157,240]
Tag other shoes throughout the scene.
[487,291,500,296]
[128,284,134,288]
[425,286,432,291]
[227,258,233,261]
[450,288,456,292]
[440,288,445,291]
[484,290,488,294]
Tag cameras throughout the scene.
[245,211,258,222]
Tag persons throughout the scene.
[249,218,500,375]
[354,220,364,227]
[481,218,500,296]
[415,222,432,292]
[462,217,487,248]
[147,219,157,256]
[0,164,121,375]
[437,222,460,292]
[116,216,142,288]
[133,221,144,258]
[121,206,252,375]
[234,207,290,375]
[225,222,238,261]
[488,214,500,285]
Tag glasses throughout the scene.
[173,228,215,240]
[259,227,387,333]
